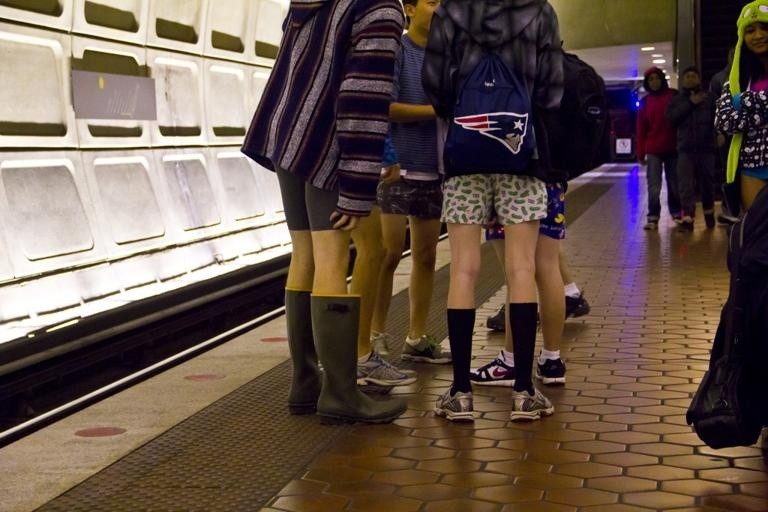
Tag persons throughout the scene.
[714,0,768,212]
[240,1,593,421]
[666,66,726,233]
[634,65,683,231]
[708,46,742,226]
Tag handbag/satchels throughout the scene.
[686,357,768,450]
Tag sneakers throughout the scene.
[643,215,658,231]
[704,210,715,229]
[717,213,741,225]
[674,216,694,232]
[355,290,591,420]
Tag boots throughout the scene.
[310,295,408,425]
[285,288,323,415]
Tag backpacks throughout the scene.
[443,49,538,177]
[560,40,611,181]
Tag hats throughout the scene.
[737,0,768,28]
